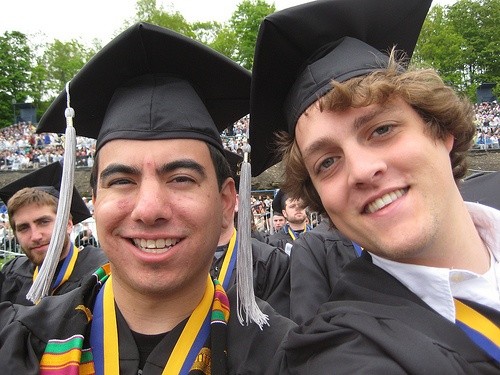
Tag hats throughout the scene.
[234,0,434,329]
[220,145,244,179]
[25,21,252,307]
[0,162,92,226]
[270,182,293,212]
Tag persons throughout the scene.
[0,161,109,306]
[0,113,330,257]
[0,21,298,375]
[468,100,500,150]
[210,149,291,319]
[248,0,500,375]
[288,170,499,327]
